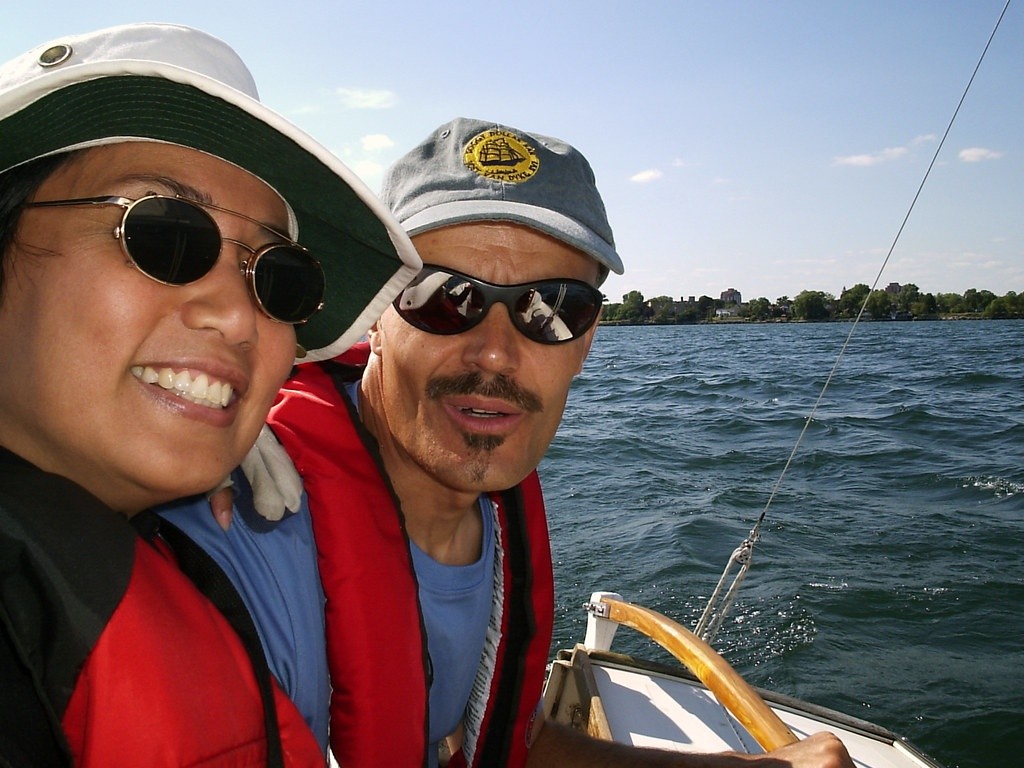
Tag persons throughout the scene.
[151,117,859,768]
[0,21,423,768]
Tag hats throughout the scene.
[382,117,625,276]
[0,21,424,366]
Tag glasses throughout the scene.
[391,262,607,344]
[8,188,327,327]
